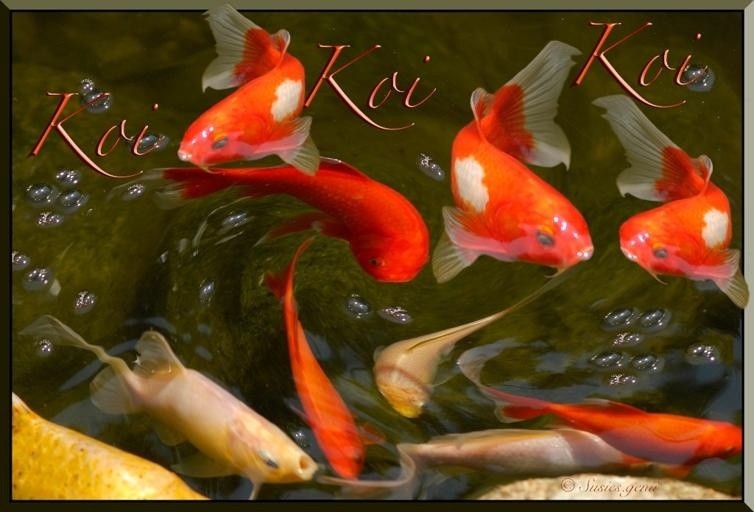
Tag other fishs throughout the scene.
[9,235,744,500]
